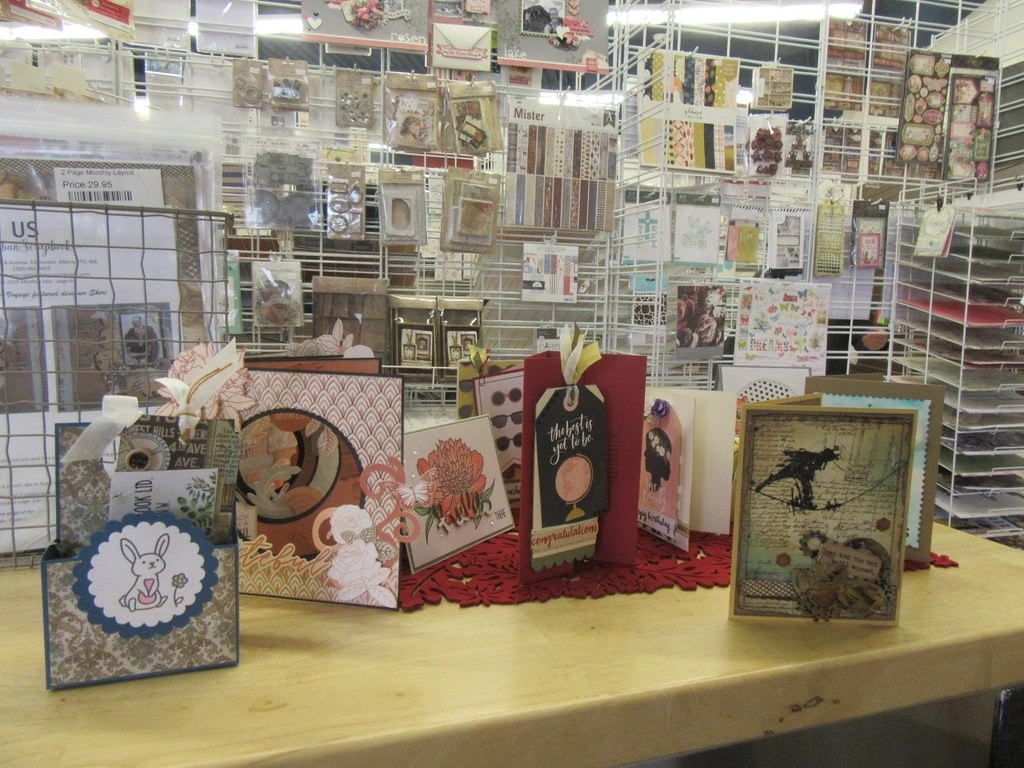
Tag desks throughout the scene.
[0,495,1024,768]
